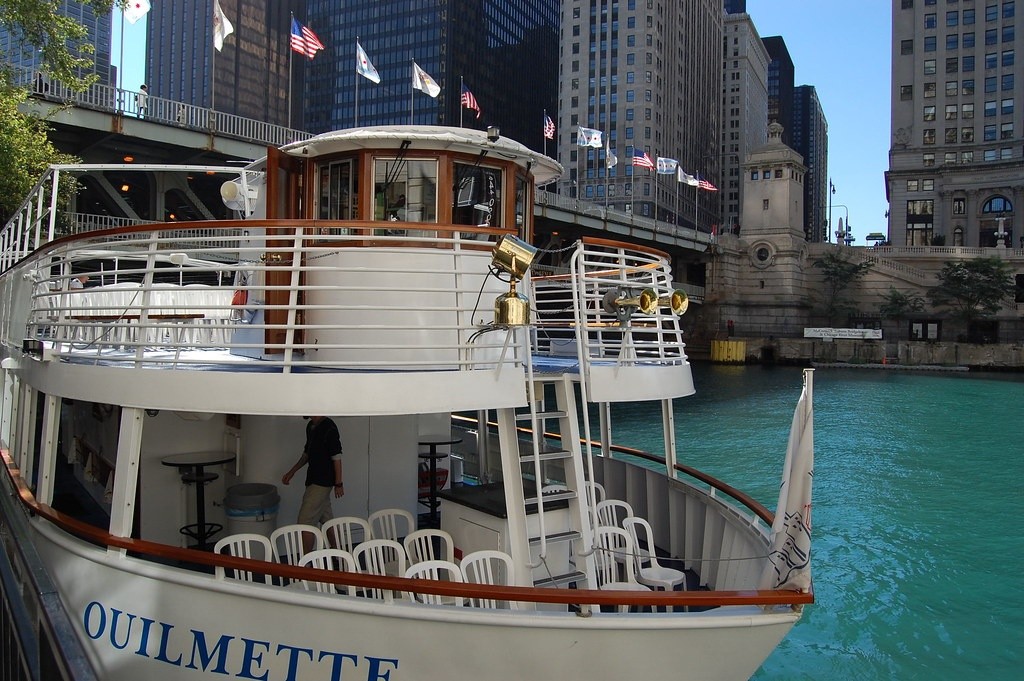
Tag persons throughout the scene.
[33,72,49,93]
[136,84,148,119]
[282,416,344,568]
[177,105,186,123]
[69,276,92,290]
[711,221,741,240]
[387,194,405,236]
[874,240,892,252]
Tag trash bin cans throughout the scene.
[221,483,281,561]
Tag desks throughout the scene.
[417,435,463,529]
[161,451,236,572]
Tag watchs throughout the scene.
[335,482,343,487]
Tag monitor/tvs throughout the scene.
[457,176,480,207]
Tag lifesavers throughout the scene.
[232,261,258,323]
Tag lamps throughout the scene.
[488,124,500,143]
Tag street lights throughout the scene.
[829,177,836,243]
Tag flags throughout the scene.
[461,85,481,119]
[678,166,698,186]
[758,392,818,590]
[577,126,603,148]
[543,116,555,139]
[413,63,441,98]
[213,0,234,52]
[697,174,718,191]
[632,149,656,172]
[356,43,381,84]
[123,0,151,25]
[657,158,678,175]
[290,11,325,62]
[606,139,618,168]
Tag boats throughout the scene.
[0,124,814,681]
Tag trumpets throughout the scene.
[613,287,689,316]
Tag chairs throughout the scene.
[32,282,235,353]
[542,480,688,613]
[214,509,520,610]
